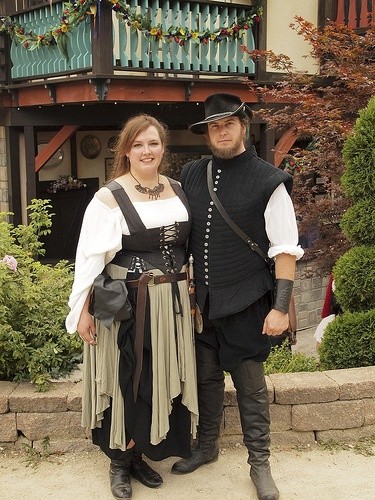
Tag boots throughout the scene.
[230,360,279,500]
[171,342,224,473]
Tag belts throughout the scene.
[125,272,193,403]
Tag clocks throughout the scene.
[80,134,102,159]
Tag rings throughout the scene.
[89,340,93,344]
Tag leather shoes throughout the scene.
[108,462,132,499]
[127,455,163,488]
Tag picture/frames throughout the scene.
[104,157,127,183]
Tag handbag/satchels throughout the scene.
[88,273,133,329]
[272,272,296,345]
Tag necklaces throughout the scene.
[129,169,165,200]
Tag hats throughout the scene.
[189,93,253,135]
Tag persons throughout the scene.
[179,94,304,500]
[66,115,199,500]
